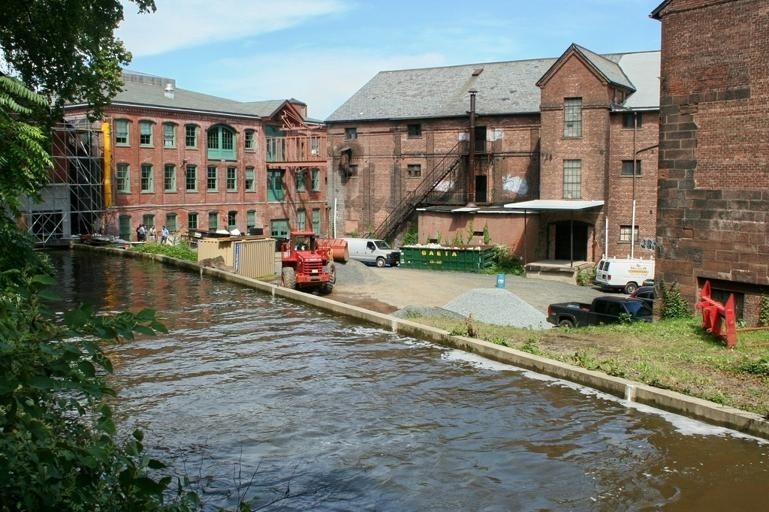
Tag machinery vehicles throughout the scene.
[279,230,337,296]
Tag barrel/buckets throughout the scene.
[496,273,505,288]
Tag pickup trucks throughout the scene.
[547,296,651,328]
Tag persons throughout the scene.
[297,240,309,249]
[136,224,169,244]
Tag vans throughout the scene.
[595,258,655,294]
[341,238,400,267]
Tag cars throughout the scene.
[630,285,654,309]
